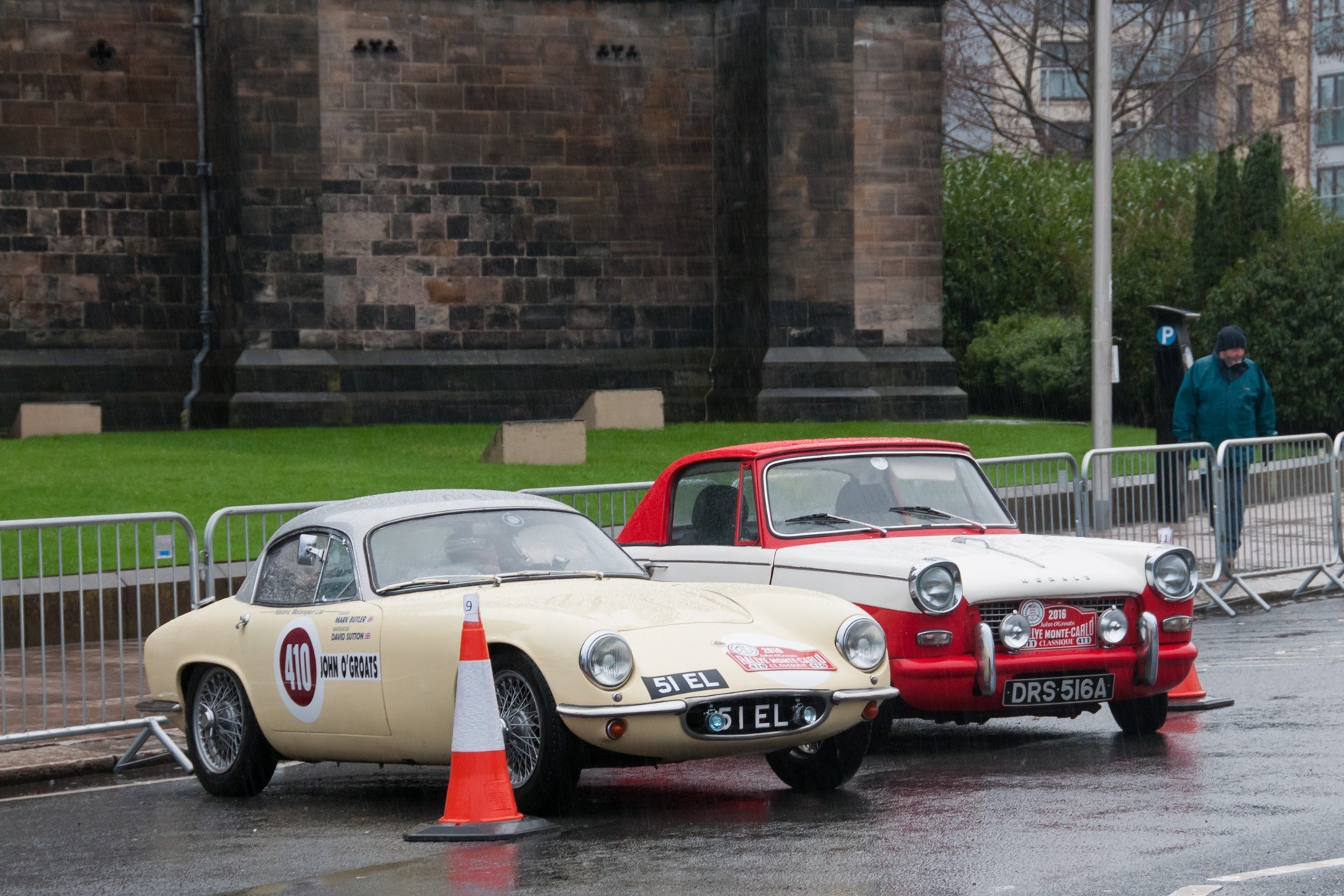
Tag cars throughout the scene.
[134,488,902,822]
[613,436,1198,748]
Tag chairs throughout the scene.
[834,479,903,531]
[444,527,530,575]
[682,484,753,545]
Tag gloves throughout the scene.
[1262,444,1274,467]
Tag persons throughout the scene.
[1172,326,1276,568]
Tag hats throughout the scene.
[1216,326,1246,352]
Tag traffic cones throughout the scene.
[1168,660,1237,711]
[403,592,565,842]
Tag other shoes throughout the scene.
[1226,555,1235,568]
